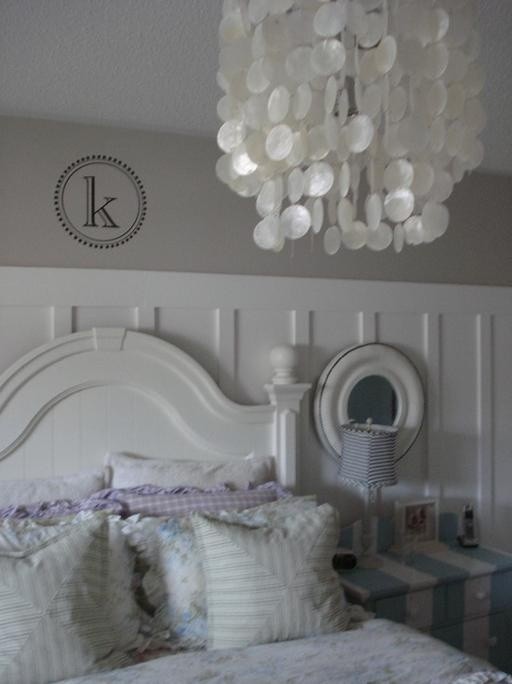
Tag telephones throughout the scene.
[457,503,479,548]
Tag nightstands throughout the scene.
[332,535,512,677]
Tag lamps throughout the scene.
[215,0,486,256]
[339,416,400,568]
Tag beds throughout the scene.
[0,326,512,684]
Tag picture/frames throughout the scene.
[393,497,439,549]
